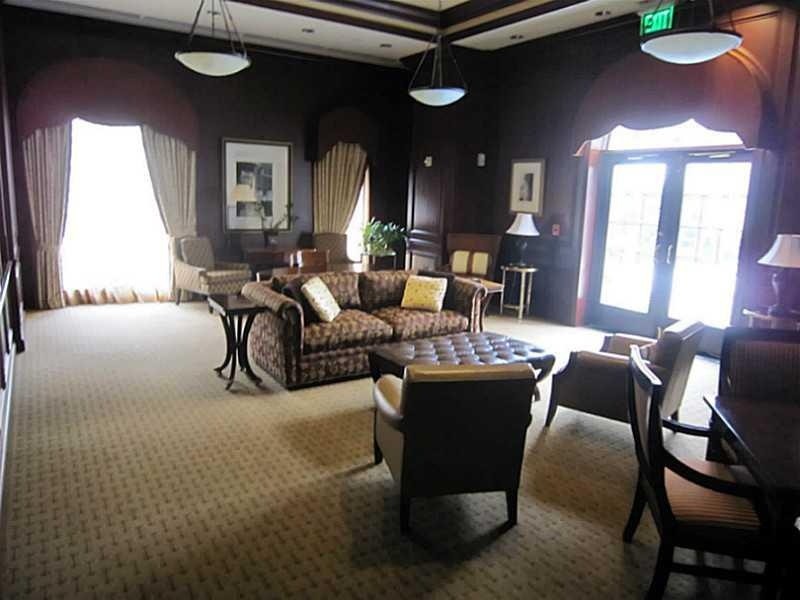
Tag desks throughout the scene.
[742,306,800,330]
[358,251,398,272]
[243,246,296,282]
[499,265,541,320]
[703,393,800,530]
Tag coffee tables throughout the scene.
[470,277,505,333]
[207,291,269,392]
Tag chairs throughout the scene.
[311,231,354,273]
[622,325,800,600]
[541,317,705,435]
[170,234,252,314]
[368,361,536,542]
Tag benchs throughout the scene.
[365,331,555,402]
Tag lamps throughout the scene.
[407,0,468,106]
[174,0,250,78]
[639,0,744,65]
[505,212,541,268]
[756,234,800,317]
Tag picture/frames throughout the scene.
[509,158,546,217]
[220,136,295,234]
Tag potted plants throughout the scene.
[362,216,410,256]
[250,200,300,250]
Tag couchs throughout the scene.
[239,269,488,392]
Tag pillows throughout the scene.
[400,269,456,313]
[282,273,342,327]
